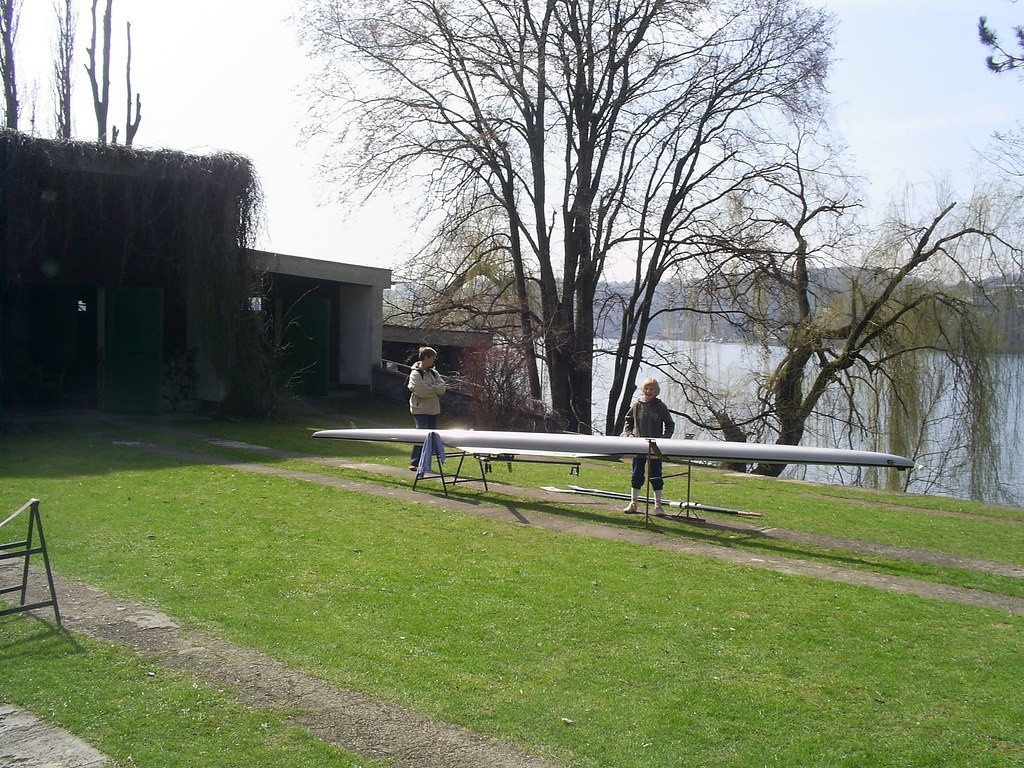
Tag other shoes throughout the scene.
[624,501,638,513]
[653,506,665,516]
[408,464,417,470]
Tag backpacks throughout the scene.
[404,368,436,402]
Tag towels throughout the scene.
[417,432,447,478]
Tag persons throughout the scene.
[407,347,445,471]
[624,378,676,517]
